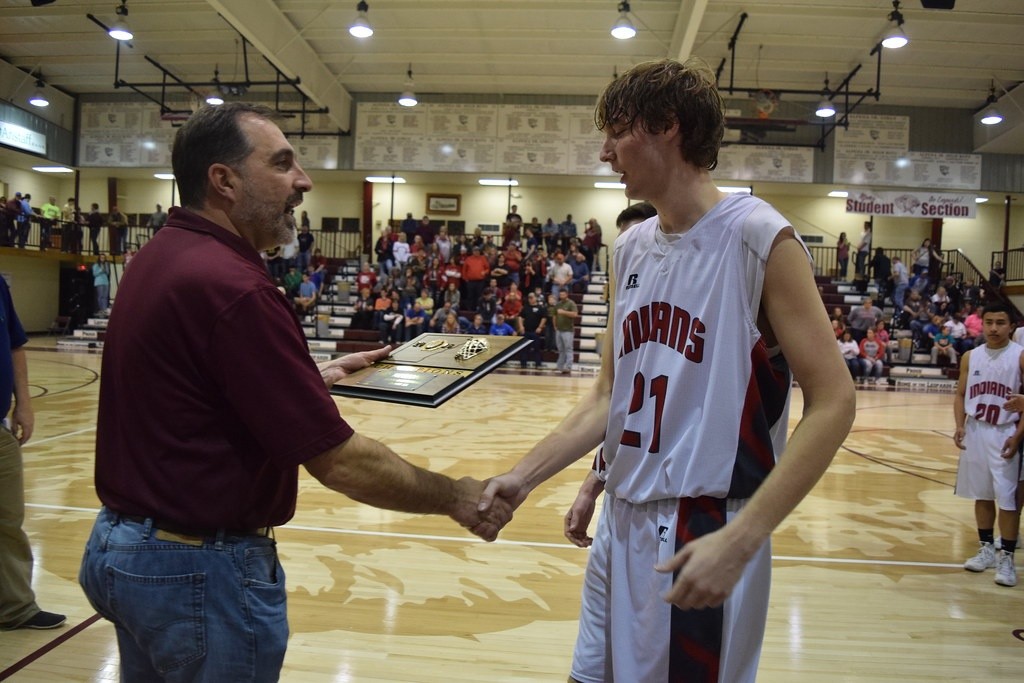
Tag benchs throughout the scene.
[791,275,962,394]
[54,263,609,377]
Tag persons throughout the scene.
[92,254,110,308]
[888,256,909,317]
[86,203,103,255]
[827,269,985,377]
[40,197,60,248]
[838,232,851,277]
[474,58,856,683]
[854,221,871,271]
[0,274,69,630]
[357,204,601,373]
[63,198,84,253]
[989,262,1004,289]
[78,99,513,683]
[869,247,891,293]
[953,302,1024,585]
[260,212,328,309]
[147,204,166,235]
[0,192,37,248]
[912,239,947,275]
[108,206,128,252]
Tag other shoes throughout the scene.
[862,380,868,385]
[20,610,67,628]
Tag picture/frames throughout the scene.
[425,192,461,217]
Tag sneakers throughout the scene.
[994,548,1016,586]
[964,541,997,571]
[994,532,1021,550]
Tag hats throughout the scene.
[472,245,479,250]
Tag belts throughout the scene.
[118,511,268,543]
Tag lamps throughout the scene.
[980,78,1005,125]
[881,0,909,49]
[205,62,225,105]
[30,66,50,107]
[815,71,837,118]
[398,61,418,108]
[108,0,134,41]
[610,0,637,40]
[348,0,373,39]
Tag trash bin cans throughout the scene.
[898,338,912,360]
[316,314,329,337]
[594,333,608,357]
[360,253,369,272]
[337,282,350,301]
